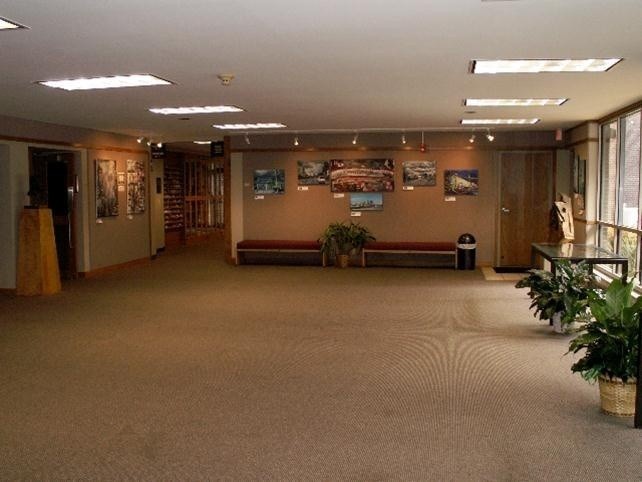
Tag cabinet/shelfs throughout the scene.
[164,164,185,251]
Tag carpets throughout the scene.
[480,266,542,281]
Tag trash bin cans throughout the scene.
[456,233,477,269]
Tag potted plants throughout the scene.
[514,257,642,418]
[316,219,376,267]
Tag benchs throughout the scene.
[236,240,327,267]
[362,241,458,269]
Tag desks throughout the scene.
[529,242,628,326]
